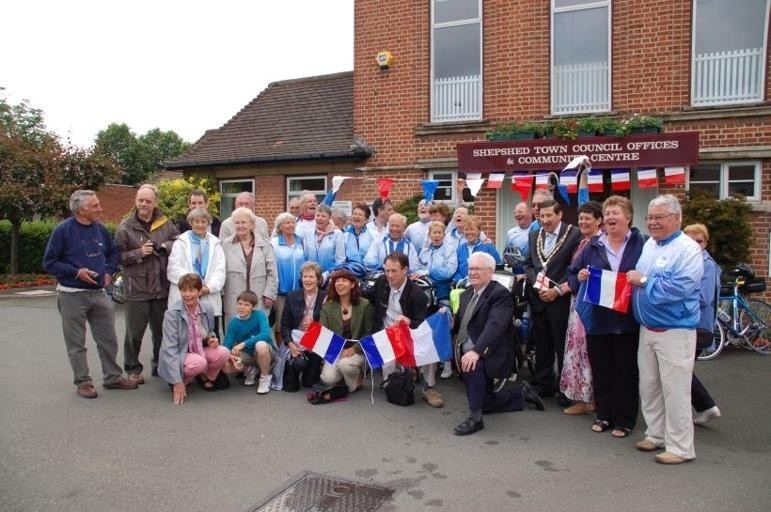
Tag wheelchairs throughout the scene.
[440,265,534,380]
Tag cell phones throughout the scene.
[90,272,99,280]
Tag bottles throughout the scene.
[521,313,529,328]
[718,308,731,322]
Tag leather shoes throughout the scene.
[128,371,147,385]
[558,390,573,407]
[353,369,366,392]
[518,378,549,411]
[531,382,556,398]
[76,382,99,399]
[103,375,139,390]
[453,414,486,436]
[149,367,161,377]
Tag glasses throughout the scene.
[645,213,677,222]
[531,202,544,209]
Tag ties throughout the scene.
[457,292,480,346]
[387,291,400,327]
[545,233,557,257]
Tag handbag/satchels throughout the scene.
[193,335,233,392]
[378,369,418,408]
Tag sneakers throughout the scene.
[634,433,667,452]
[655,447,697,464]
[420,385,445,408]
[692,404,724,427]
[562,399,598,416]
[255,374,274,395]
[195,371,217,391]
[440,365,456,379]
[244,365,261,386]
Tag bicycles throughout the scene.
[696,264,771,360]
[109,258,126,304]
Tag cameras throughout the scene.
[151,240,167,257]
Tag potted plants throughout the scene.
[543,114,599,139]
[483,120,542,142]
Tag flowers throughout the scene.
[605,112,663,138]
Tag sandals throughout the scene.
[591,414,613,433]
[611,422,634,439]
